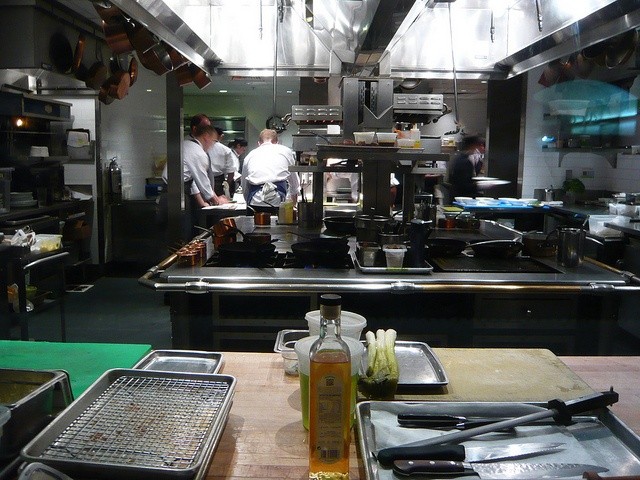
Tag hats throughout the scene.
[234,137,248,147]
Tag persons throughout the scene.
[207,128,235,227]
[445,136,495,202]
[468,137,486,178]
[225,139,248,192]
[152,126,219,265]
[185,114,211,140]
[242,128,298,216]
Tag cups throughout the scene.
[305,310,367,341]
[294,335,364,434]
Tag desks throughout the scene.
[0,243,69,341]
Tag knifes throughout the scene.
[393,458,611,480]
[377,442,568,469]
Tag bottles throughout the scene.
[307,294,352,480]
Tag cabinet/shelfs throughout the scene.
[0,91,73,212]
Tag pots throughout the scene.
[247,203,272,225]
[471,238,525,260]
[353,207,390,241]
[522,227,560,258]
[228,223,272,246]
[292,244,350,267]
[193,218,237,248]
[168,238,207,266]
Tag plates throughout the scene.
[10,190,39,209]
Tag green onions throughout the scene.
[374,329,386,391]
[384,328,399,387]
[360,330,376,390]
